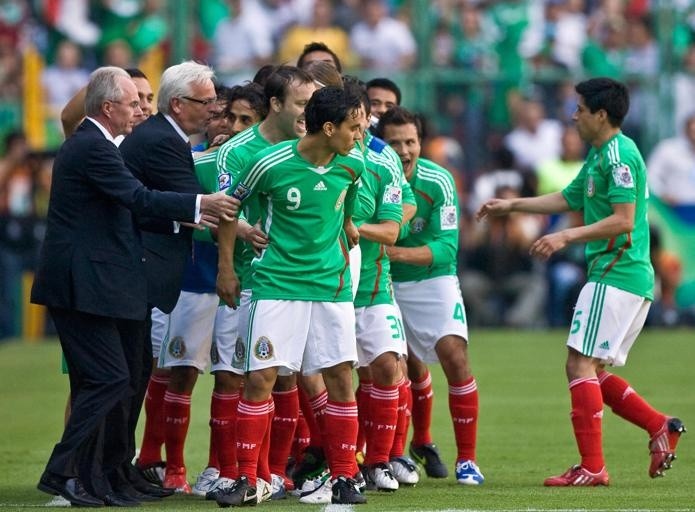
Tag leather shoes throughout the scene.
[35,466,175,507]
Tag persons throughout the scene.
[0,0,695,336]
[472,79,688,488]
[32,41,481,511]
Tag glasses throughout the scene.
[181,96,217,106]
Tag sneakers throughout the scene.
[135,445,486,507]
[648,416,689,479]
[543,464,611,487]
